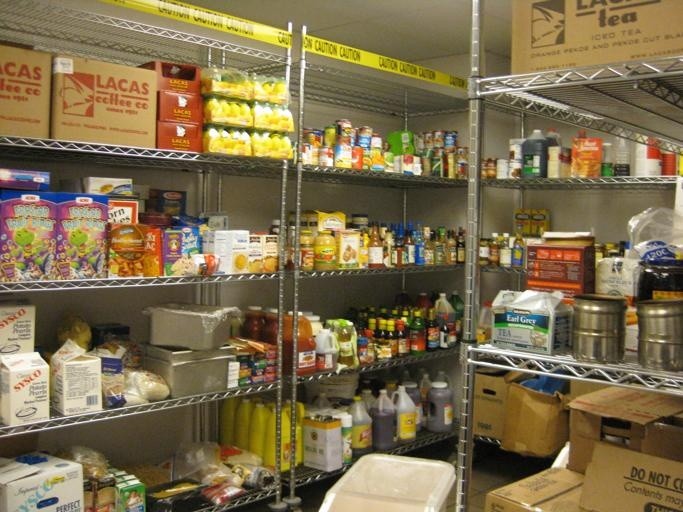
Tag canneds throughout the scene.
[270,219,337,272]
[292,119,522,179]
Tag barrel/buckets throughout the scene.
[521,127,561,179]
[218,369,455,473]
[395,288,492,347]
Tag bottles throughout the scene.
[271,218,336,272]
[325,287,465,366]
[488,232,525,269]
[367,219,465,270]
[546,125,631,178]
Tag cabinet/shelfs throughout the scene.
[0,0,526,512]
[455,0,683,510]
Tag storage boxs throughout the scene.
[143,304,239,352]
[510,0,682,77]
[0,453,84,512]
[50,51,158,147]
[140,341,237,399]
[316,453,456,512]
[0,45,52,138]
[471,371,683,512]
[490,231,639,359]
